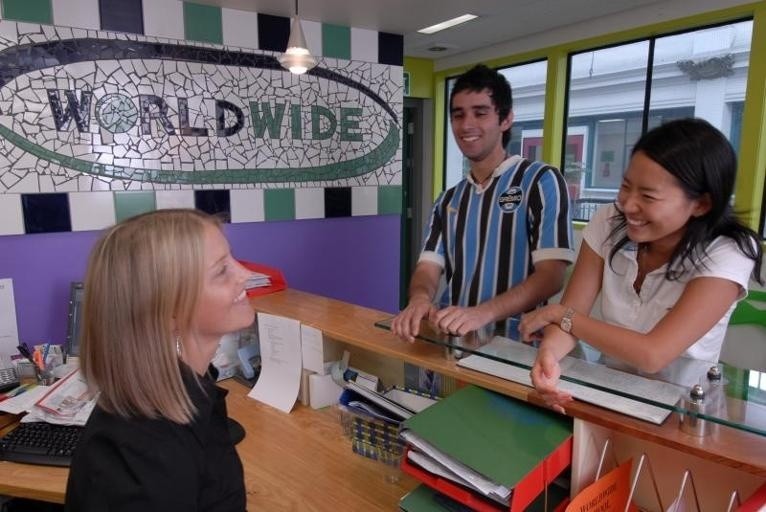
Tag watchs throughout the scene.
[561,307,573,332]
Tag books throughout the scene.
[398,383,573,512]
[337,389,402,428]
[345,379,415,422]
[456,335,689,426]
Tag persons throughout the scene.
[518,117,765,416]
[63,207,257,511]
[389,63,574,351]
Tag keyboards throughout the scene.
[0,422,87,467]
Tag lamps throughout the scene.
[279,2,319,74]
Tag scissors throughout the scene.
[16,335,44,373]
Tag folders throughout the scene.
[398,383,573,511]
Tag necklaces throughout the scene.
[471,150,510,196]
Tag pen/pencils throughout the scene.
[43,338,52,363]
